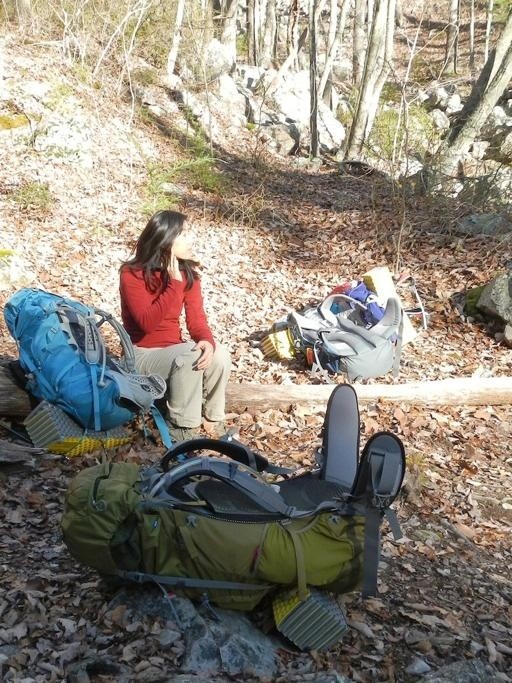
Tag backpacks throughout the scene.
[4,289,176,448]
[288,293,401,379]
[61,384,405,611]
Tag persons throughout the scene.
[118,208,233,453]
